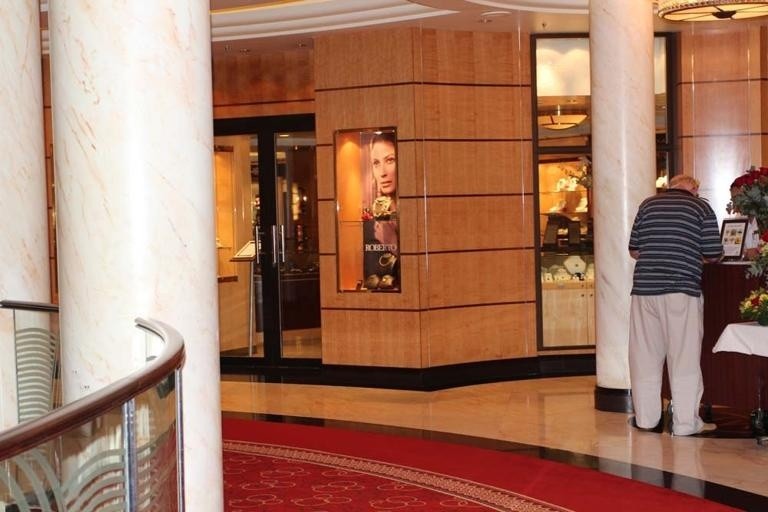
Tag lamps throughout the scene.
[658,0,768,22]
[537,108,588,130]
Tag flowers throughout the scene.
[725,165,768,325]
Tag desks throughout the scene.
[712,321,768,445]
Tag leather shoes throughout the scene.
[695,422,717,433]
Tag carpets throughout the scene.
[26,416,741,512]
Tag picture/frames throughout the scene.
[719,218,749,259]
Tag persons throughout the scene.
[627,174,720,436]
[367,133,397,216]
[371,220,399,287]
[542,182,588,238]
